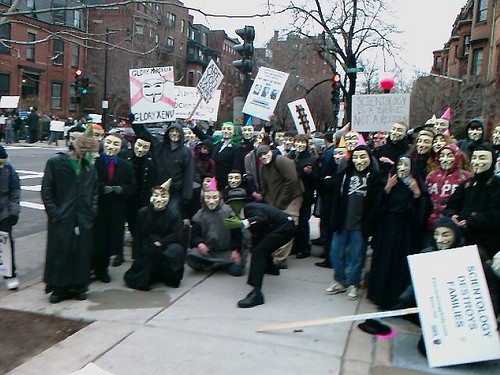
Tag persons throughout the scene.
[0,144,21,288]
[0,109,76,147]
[40,116,500,359]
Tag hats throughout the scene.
[225,188,254,205]
[256,145,271,157]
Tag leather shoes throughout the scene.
[315,260,334,268]
[238,290,264,308]
[311,237,326,245]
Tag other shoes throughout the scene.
[6,278,20,290]
[75,292,87,300]
[114,255,124,266]
[50,290,64,302]
[94,270,110,283]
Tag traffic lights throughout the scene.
[331,74,341,104]
[75,69,82,90]
[81,77,89,95]
[233,25,255,73]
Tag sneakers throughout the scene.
[326,282,347,294]
[347,285,358,300]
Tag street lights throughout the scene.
[101,26,132,129]
